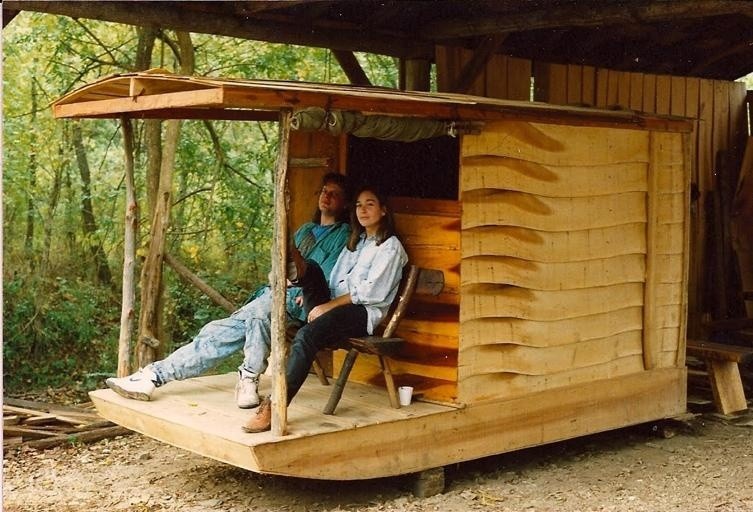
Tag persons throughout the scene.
[102,169,356,412]
[238,179,409,435]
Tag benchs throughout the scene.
[687,340,752,415]
[284,258,444,417]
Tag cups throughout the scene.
[398,386,413,405]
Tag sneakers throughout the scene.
[235,369,261,408]
[104,366,158,402]
[242,397,273,433]
[286,228,307,284]
